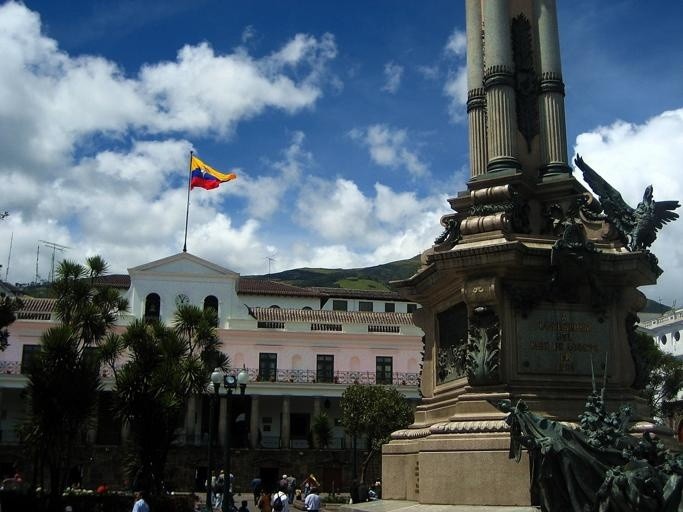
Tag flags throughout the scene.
[190,151,236,191]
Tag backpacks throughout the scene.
[272,492,285,511]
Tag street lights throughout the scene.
[206,365,248,511]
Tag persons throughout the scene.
[204,468,251,512]
[251,473,322,512]
[97,480,109,495]
[328,477,382,503]
[132,491,150,512]
